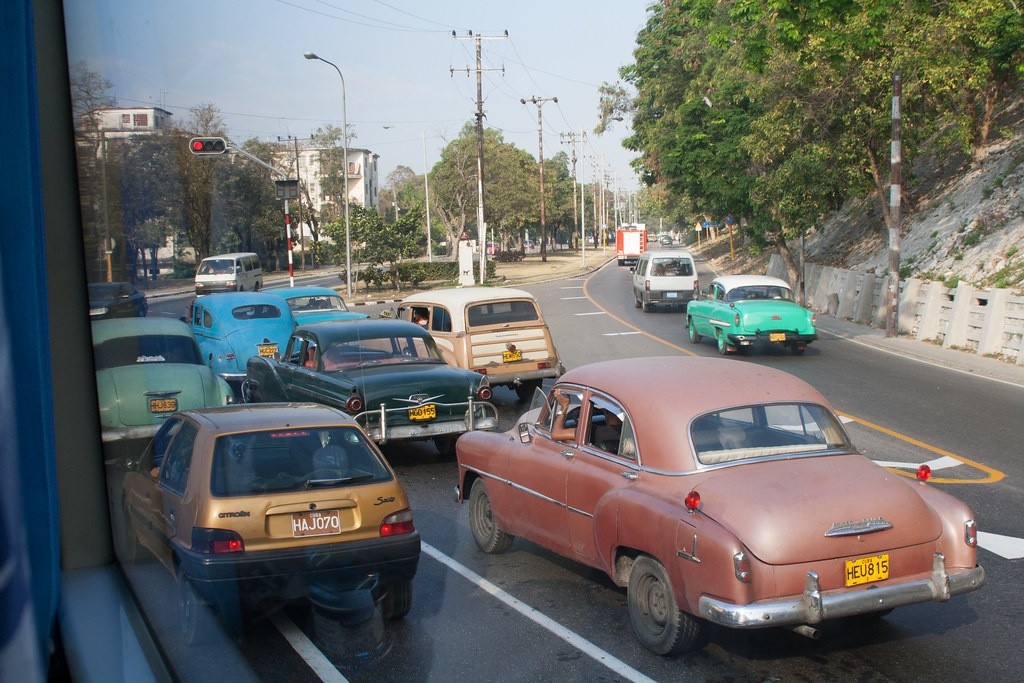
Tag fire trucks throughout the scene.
[616,223,648,266]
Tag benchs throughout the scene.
[595,426,751,455]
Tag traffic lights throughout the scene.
[275,181,301,200]
[190,137,226,155]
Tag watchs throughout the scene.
[557,410,566,416]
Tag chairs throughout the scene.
[305,346,346,368]
[698,444,828,466]
[313,446,349,471]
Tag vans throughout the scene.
[195,252,263,297]
[629,250,700,312]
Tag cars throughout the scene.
[87,282,149,320]
[378,286,567,400]
[90,317,240,442]
[487,242,502,255]
[523,240,535,249]
[121,407,422,619]
[180,292,319,382]
[684,274,820,355]
[453,355,987,658]
[648,235,673,246]
[241,318,497,453]
[587,236,601,243]
[256,287,371,348]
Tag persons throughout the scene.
[151,466,169,479]
[551,388,622,441]
[414,307,430,325]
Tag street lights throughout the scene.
[304,51,355,297]
[580,117,625,268]
[383,125,433,263]
